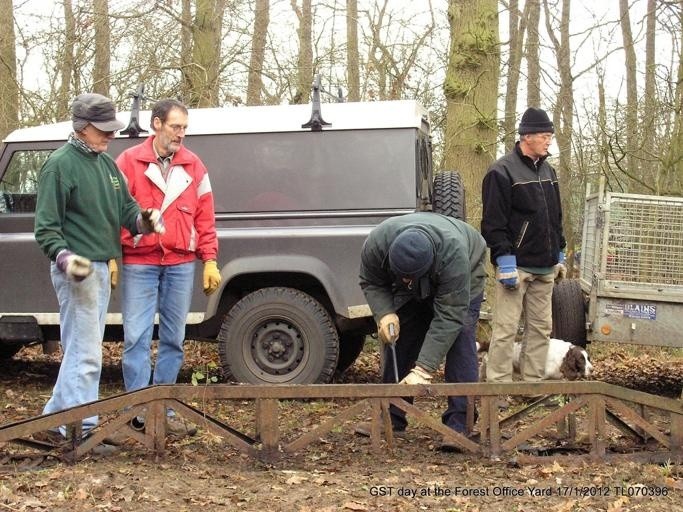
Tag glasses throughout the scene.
[164,122,188,134]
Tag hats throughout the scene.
[70,91,127,134]
[518,107,555,135]
[387,227,435,280]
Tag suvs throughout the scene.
[0,71,468,386]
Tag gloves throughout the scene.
[107,258,119,291]
[377,311,401,346]
[135,208,165,237]
[494,254,521,291]
[53,249,94,283]
[398,364,433,386]
[202,259,221,297]
[554,247,567,286]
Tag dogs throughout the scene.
[475,338,594,402]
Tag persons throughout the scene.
[355,210,488,452]
[479,106,567,409]
[32,92,165,446]
[108,99,221,436]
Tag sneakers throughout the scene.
[355,418,394,437]
[33,414,198,458]
[511,395,561,408]
[498,398,508,411]
[439,432,470,453]
